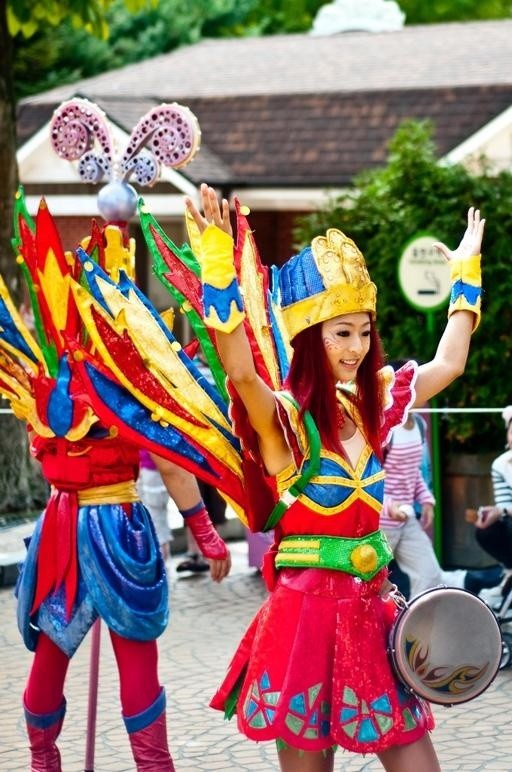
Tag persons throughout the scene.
[135,443,174,569]
[175,474,228,573]
[464,404,512,573]
[377,357,444,603]
[3,225,233,771]
[182,180,486,771]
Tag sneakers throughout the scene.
[174,550,211,574]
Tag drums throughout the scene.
[390,586,502,707]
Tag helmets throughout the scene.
[274,231,379,343]
